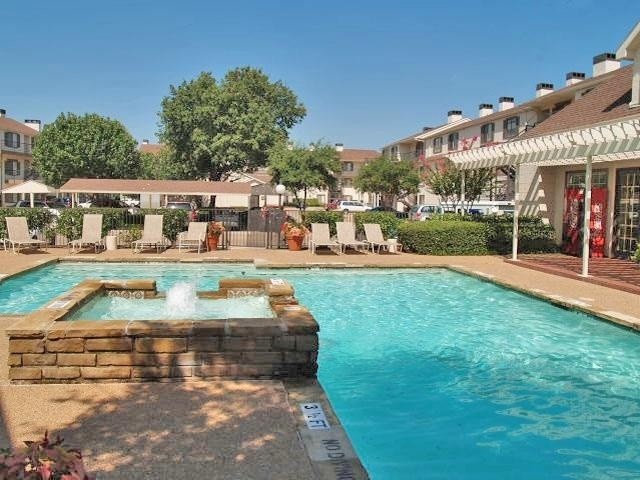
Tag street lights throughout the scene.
[274,182,287,251]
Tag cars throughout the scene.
[14,195,249,231]
[326,199,514,222]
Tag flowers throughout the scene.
[207,221,225,240]
[0,428,89,480]
[280,220,307,241]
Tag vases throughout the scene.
[208,239,217,251]
[288,239,303,251]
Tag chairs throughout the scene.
[69,213,106,253]
[131,215,208,254]
[308,221,402,256]
[4,217,48,256]
